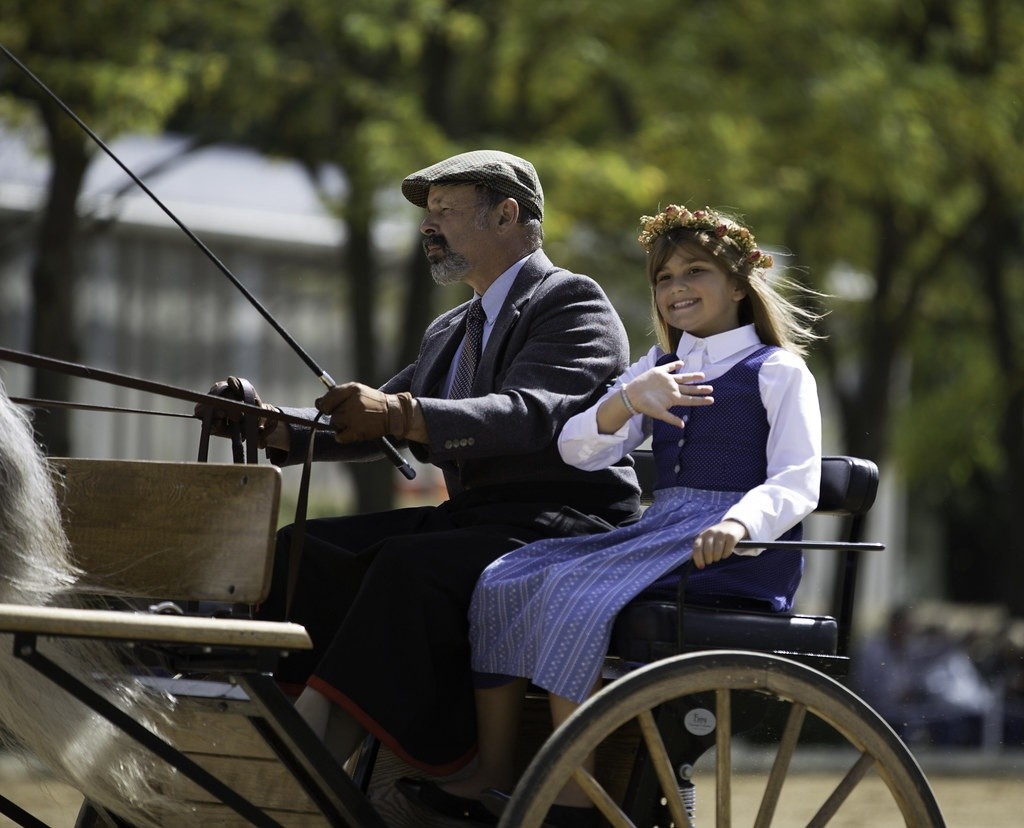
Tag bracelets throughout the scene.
[620,383,640,416]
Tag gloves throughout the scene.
[315,381,413,444]
[195,376,278,440]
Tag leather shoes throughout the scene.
[394,775,604,827]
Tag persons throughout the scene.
[195,153,641,710]
[863,605,987,753]
[395,206,821,828]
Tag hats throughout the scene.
[401,150,545,224]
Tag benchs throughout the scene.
[601,447,889,660]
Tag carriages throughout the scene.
[0,46,959,827]
